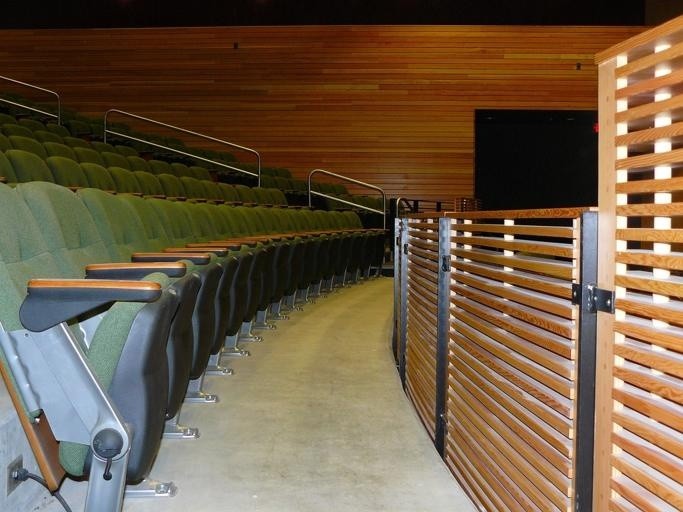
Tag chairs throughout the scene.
[0,95,412,512]
